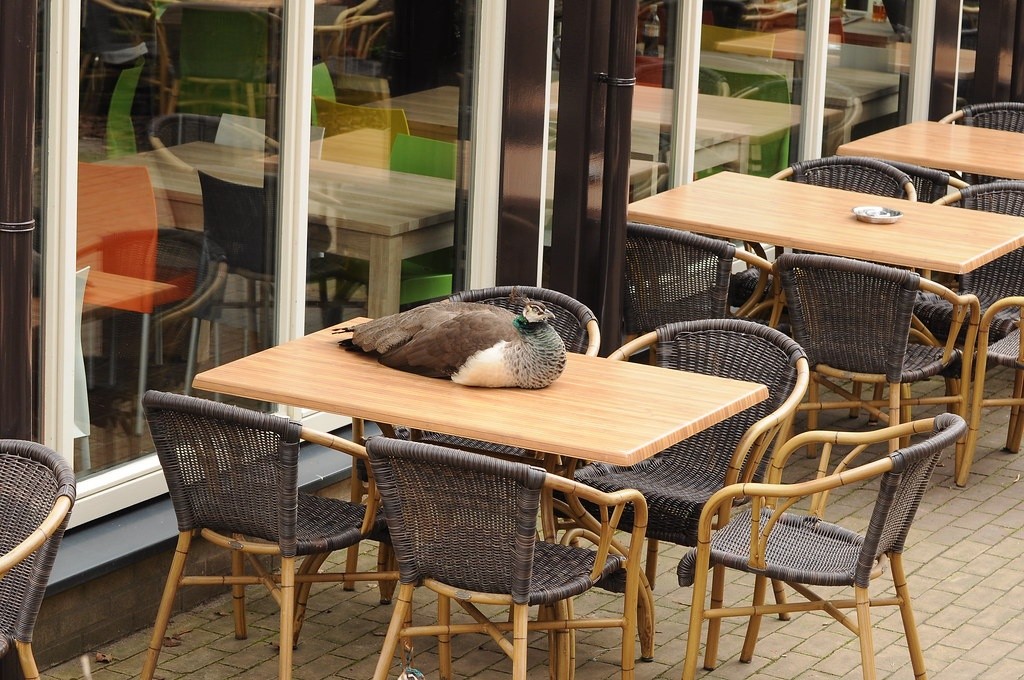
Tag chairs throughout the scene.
[0,0,1023,680]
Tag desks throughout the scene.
[619,167,1024,282]
[784,65,901,146]
[836,119,1023,189]
[717,30,977,84]
[90,139,458,323]
[195,316,769,660]
[258,123,670,215]
[359,86,839,204]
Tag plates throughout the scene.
[851,207,903,223]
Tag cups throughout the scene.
[873,0,887,22]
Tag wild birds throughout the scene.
[330,285,568,391]
